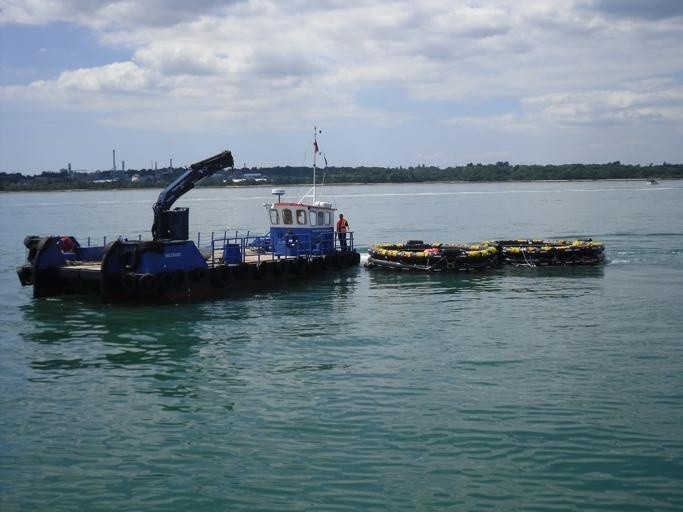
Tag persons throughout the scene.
[337,214,349,252]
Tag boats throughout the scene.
[486,237,605,268]
[363,239,500,274]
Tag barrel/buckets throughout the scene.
[222,244,244,263]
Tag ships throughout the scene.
[17,126,360,308]
